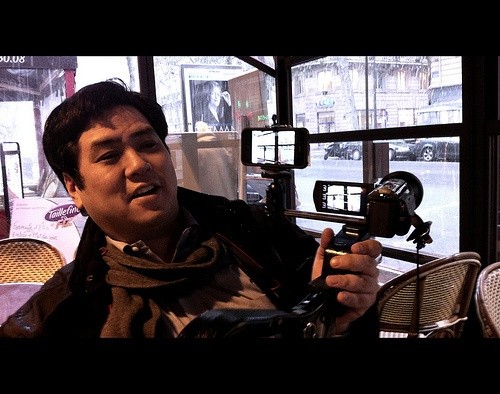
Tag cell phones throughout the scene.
[241,127,310,170]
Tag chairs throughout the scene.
[376,252,481,339]
[0,238,65,284]
[474,261,500,338]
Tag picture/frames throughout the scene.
[182,65,245,133]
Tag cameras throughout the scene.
[313,171,424,238]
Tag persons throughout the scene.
[194,81,232,131]
[0,78,384,394]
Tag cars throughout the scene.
[323,136,460,162]
